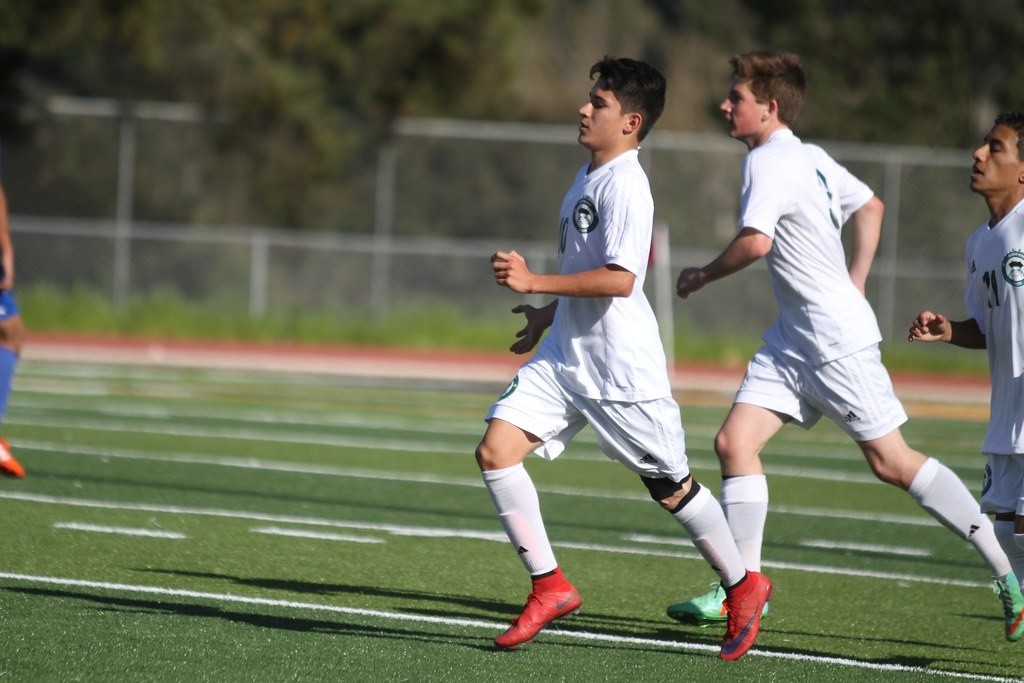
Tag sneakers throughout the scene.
[667,582,770,628]
[719,570,774,660]
[991,571,1024,641]
[0,438,25,478]
[493,580,582,650]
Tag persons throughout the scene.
[0,187,24,479]
[476,58,772,660]
[909,109,1024,596]
[667,50,1023,641]
